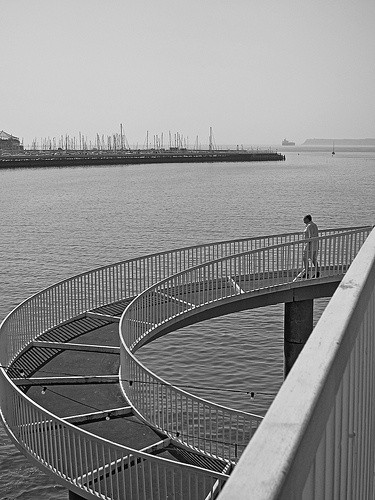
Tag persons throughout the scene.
[301,215,320,278]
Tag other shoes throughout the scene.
[312,271,320,278]
[298,273,310,279]
